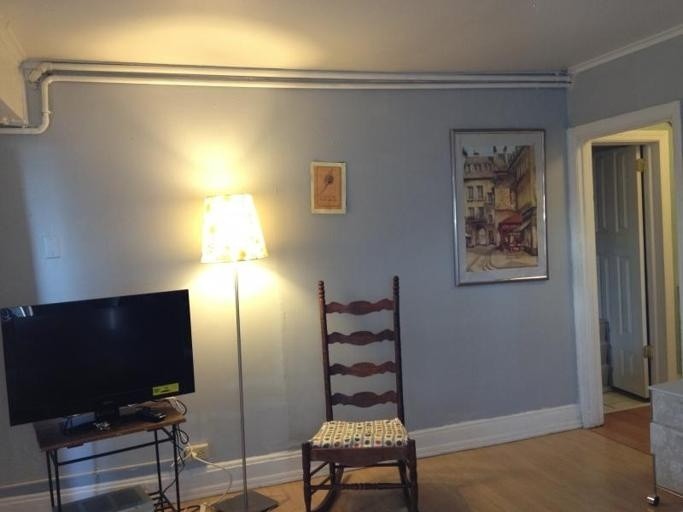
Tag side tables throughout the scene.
[33,400,187,512]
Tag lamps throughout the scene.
[201,192,282,511]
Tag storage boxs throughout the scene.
[645,377,683,506]
[601,340,610,365]
[599,318,609,341]
[602,363,611,387]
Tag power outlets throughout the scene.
[184,443,210,468]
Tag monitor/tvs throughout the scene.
[1,286,197,428]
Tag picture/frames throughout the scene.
[308,159,347,217]
[448,127,549,287]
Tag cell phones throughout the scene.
[133,407,166,423]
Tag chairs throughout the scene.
[302,275,418,511]
